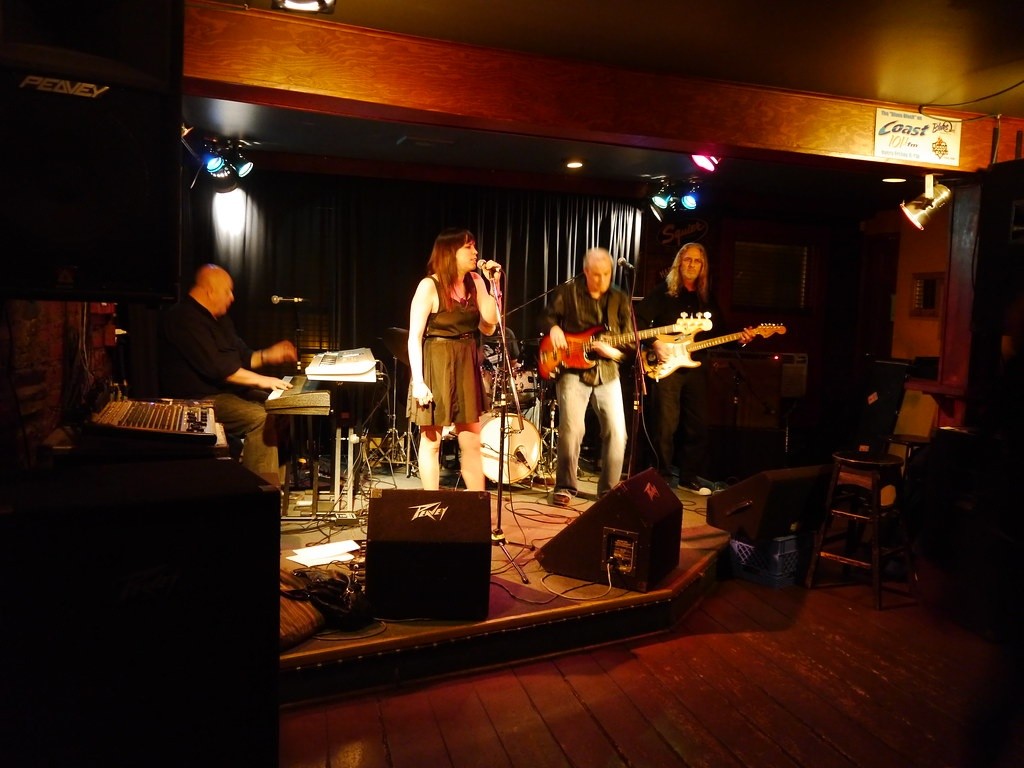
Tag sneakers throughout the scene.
[678,478,711,495]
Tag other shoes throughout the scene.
[553,488,572,505]
[597,492,608,500]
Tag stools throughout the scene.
[805,434,930,608]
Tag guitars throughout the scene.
[537,311,714,383]
[640,322,788,382]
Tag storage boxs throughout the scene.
[728,540,804,589]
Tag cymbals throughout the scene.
[520,338,542,345]
[483,335,513,344]
[383,326,412,367]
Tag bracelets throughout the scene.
[260,349,264,366]
[489,292,502,298]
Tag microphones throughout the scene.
[516,451,531,470]
[272,295,305,304]
[477,259,502,272]
[618,257,635,270]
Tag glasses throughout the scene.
[683,258,702,264]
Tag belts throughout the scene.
[426,332,474,340]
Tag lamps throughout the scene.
[198,138,253,178]
[898,173,958,230]
[639,155,725,223]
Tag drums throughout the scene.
[504,366,538,393]
[480,366,495,399]
[478,409,542,485]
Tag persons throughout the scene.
[406,227,502,492]
[153,264,305,505]
[637,242,756,496]
[540,249,639,505]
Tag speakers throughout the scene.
[706,465,832,546]
[365,488,492,621]
[975,158,1024,284]
[702,423,819,486]
[0,457,279,768]
[0,0,185,306]
[534,466,683,594]
[704,349,823,430]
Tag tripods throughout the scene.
[541,399,584,477]
[360,352,422,479]
[486,268,535,584]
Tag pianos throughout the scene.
[265,346,378,519]
